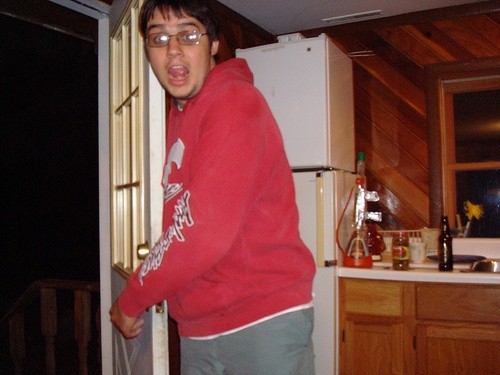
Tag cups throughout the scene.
[410,242,426,264]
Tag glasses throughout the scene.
[146,30,207,47]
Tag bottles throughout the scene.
[364,219,386,255]
[438,216,454,272]
[357,152,365,175]
[354,177,367,231]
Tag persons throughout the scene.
[456,170,500,236]
[108,0,316,375]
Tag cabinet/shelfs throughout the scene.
[337,277,500,375]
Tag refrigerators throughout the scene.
[235,33,357,375]
